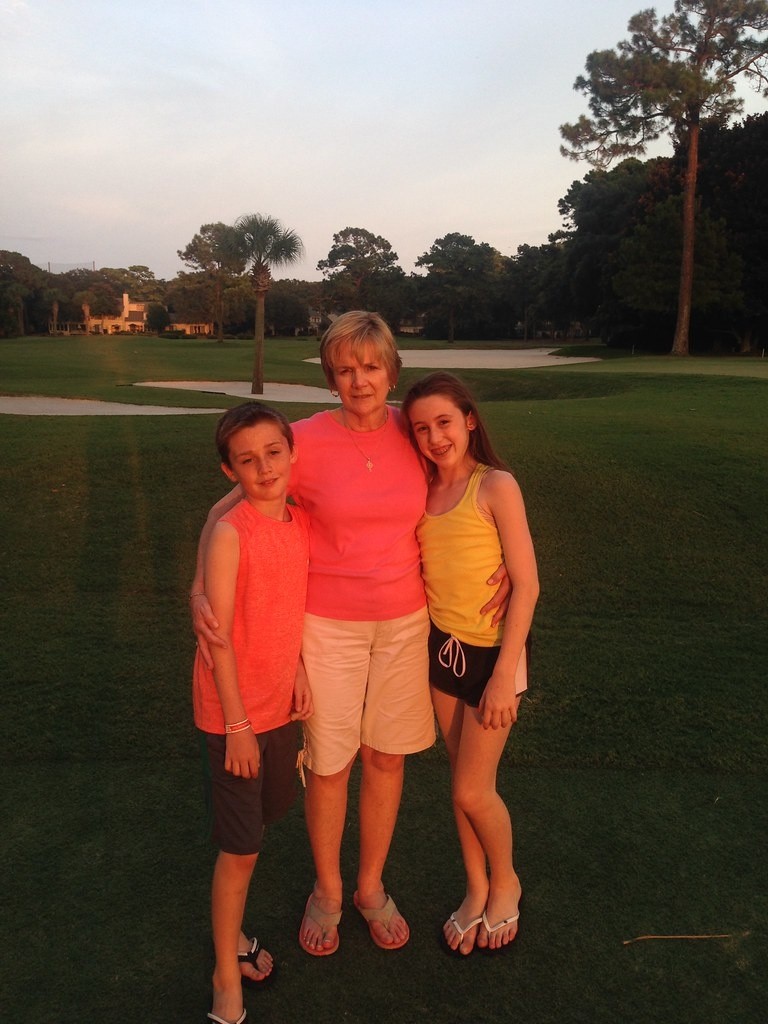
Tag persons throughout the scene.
[189,312,540,1024]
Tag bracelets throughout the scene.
[226,718,251,733]
[190,592,204,598]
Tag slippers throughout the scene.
[299,892,343,956]
[440,911,482,960]
[204,1007,251,1024]
[353,887,410,949]
[477,910,521,949]
[237,935,278,988]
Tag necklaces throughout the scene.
[341,406,388,472]
